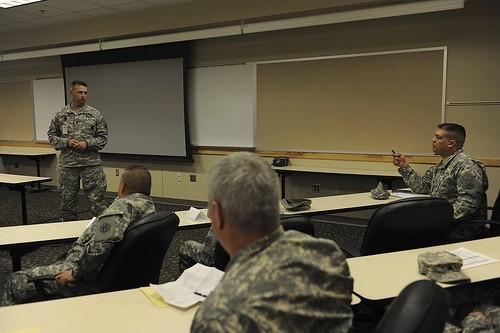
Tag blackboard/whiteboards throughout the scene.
[31,62,257,151]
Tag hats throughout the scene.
[281,198,311,211]
[371,181,391,200]
[418,249,471,283]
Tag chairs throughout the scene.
[451,184,500,243]
[28,211,179,304]
[370,278,452,333]
[340,196,454,259]
[278,216,318,240]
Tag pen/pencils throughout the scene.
[194,292,207,297]
[392,150,395,154]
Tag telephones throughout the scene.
[273,156,289,167]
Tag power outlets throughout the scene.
[177,175,182,183]
[115,169,119,176]
[333,185,340,194]
[14,162,19,168]
[312,183,322,193]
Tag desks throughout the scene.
[280,187,431,219]
[0,146,56,194]
[272,165,402,199]
[0,289,201,333]
[0,205,211,274]
[0,172,53,225]
[346,235,500,328]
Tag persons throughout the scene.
[181,227,218,266]
[443,279,500,333]
[2,165,157,307]
[392,123,487,246]
[47,80,109,221]
[190,151,355,333]
